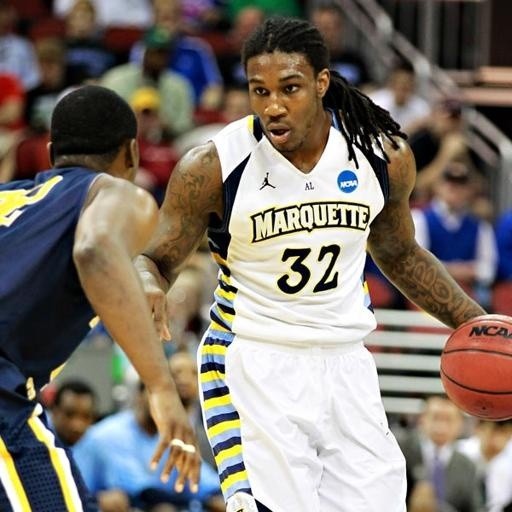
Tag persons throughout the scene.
[1,0,512,511]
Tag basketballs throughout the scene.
[440,314,512,422]
